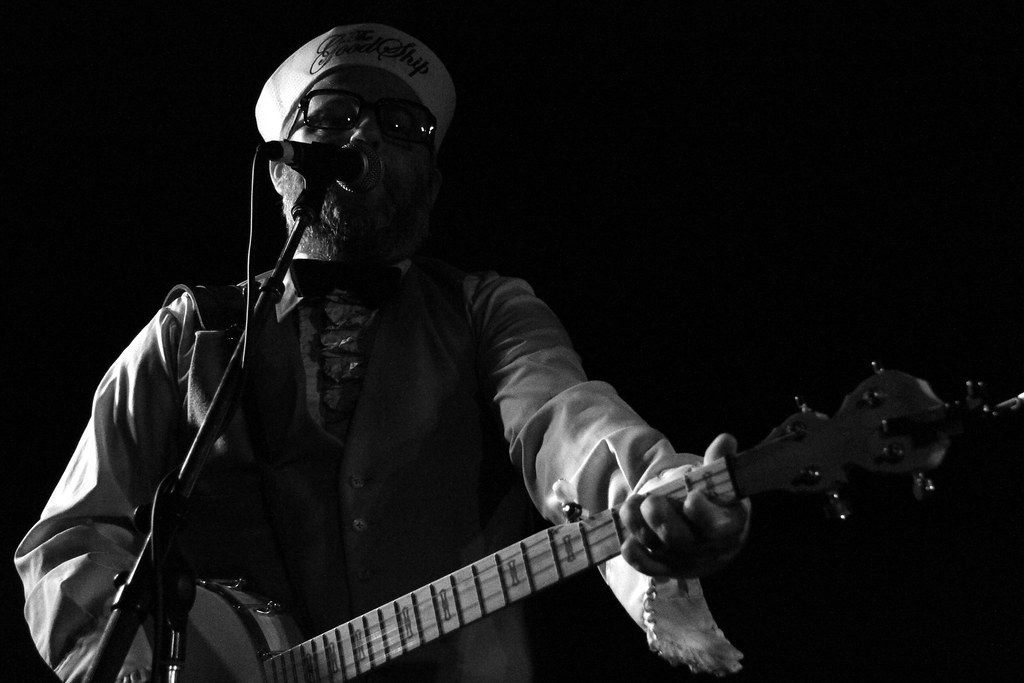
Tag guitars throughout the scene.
[183,363,1023,683]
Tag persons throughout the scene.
[12,25,752,683]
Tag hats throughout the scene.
[252,23,457,152]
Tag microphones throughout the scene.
[260,142,388,193]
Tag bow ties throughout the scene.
[290,257,401,306]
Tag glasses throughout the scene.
[282,89,436,145]
